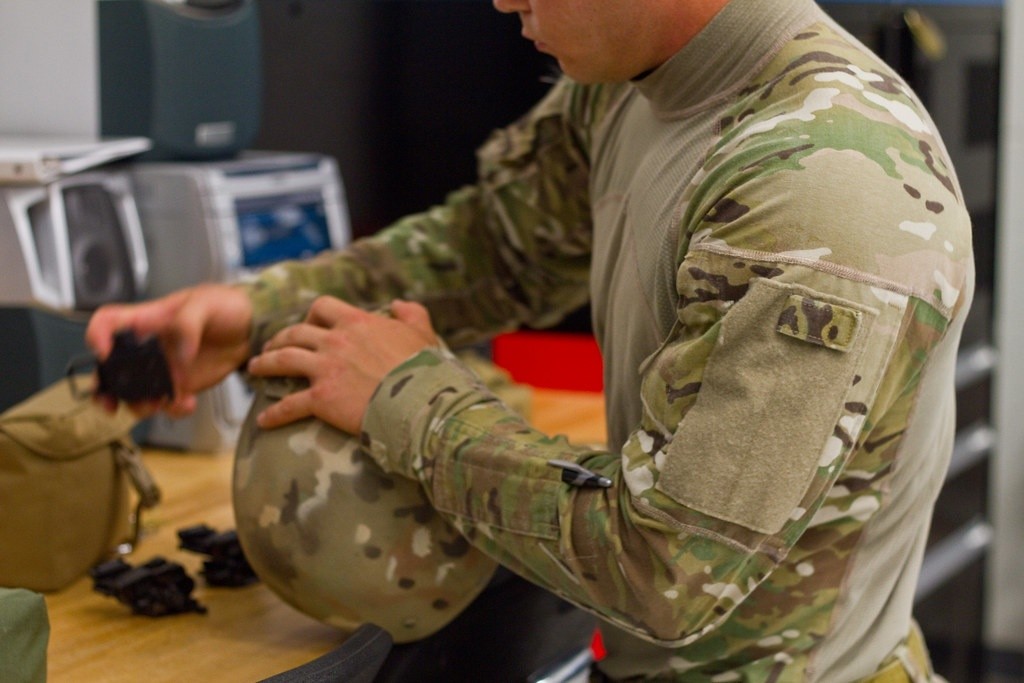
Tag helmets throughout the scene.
[235,314,500,643]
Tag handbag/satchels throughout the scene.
[0,372,161,595]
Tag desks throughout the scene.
[0,382,615,682]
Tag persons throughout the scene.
[80,0,978,683]
